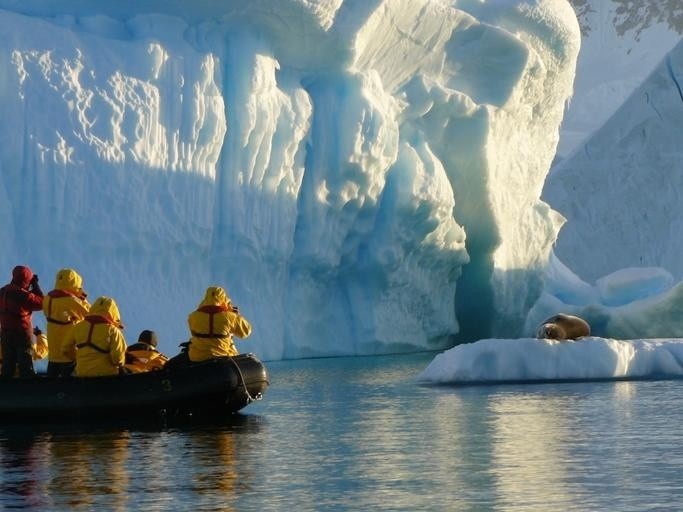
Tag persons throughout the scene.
[124,328,170,374]
[43,268,90,376]
[13,324,48,374]
[185,285,252,365]
[60,296,126,378]
[0,263,44,376]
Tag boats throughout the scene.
[1,351,270,429]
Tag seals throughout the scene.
[536,313,590,341]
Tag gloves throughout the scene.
[32,274,39,284]
[33,326,41,335]
